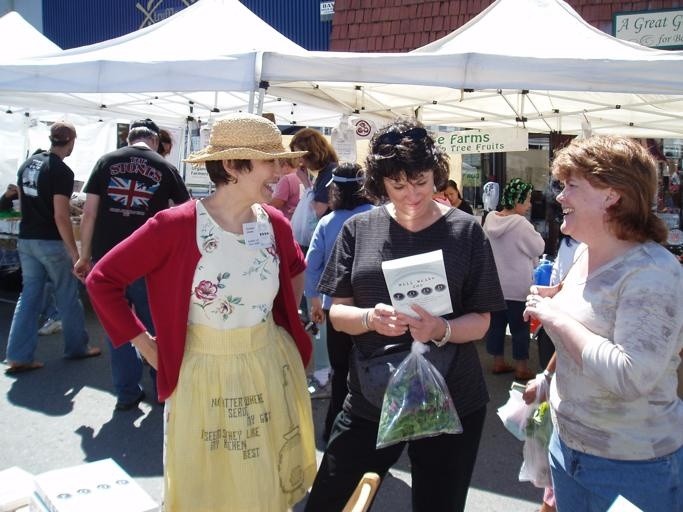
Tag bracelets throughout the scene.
[431,318,451,347]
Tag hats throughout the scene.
[181,112,309,163]
[50,120,77,142]
[132,118,165,153]
[325,174,363,188]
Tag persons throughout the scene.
[269,130,377,443]
[85,116,318,512]
[522,136,682,511]
[0,118,191,407]
[443,181,582,382]
[303,120,505,511]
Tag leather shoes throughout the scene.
[515,368,536,381]
[492,365,513,374]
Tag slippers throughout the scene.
[6,361,45,375]
[87,347,102,356]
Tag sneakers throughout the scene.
[39,319,63,335]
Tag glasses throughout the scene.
[372,127,432,153]
